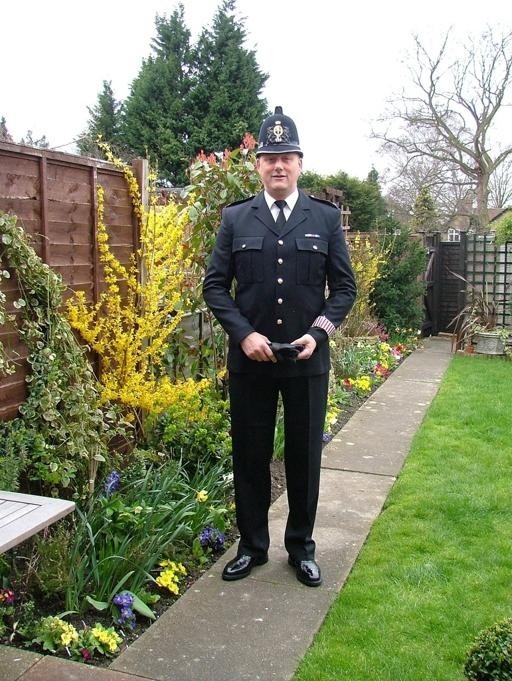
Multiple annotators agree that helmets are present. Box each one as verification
[256,106,303,158]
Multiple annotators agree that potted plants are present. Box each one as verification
[442,262,512,356]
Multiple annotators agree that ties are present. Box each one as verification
[275,200,287,232]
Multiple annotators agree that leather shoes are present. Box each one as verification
[288,554,322,586]
[222,553,267,581]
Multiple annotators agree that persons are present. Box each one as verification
[203,104,358,587]
